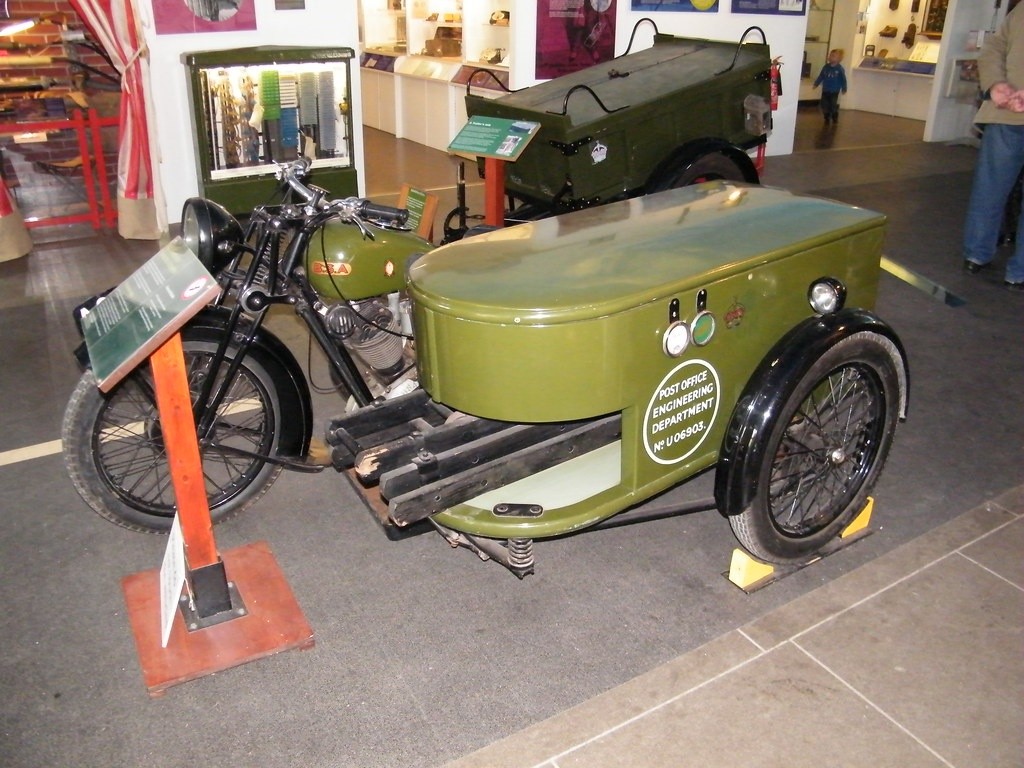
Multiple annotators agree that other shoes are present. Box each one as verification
[965,258,992,273]
[832,114,838,124]
[1004,277,1024,292]
[824,116,830,124]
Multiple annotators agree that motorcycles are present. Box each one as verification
[61,18,912,566]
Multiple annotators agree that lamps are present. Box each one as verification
[0,16,120,81]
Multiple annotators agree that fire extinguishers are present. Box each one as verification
[770,55,784,111]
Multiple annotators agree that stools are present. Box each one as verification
[36,153,106,215]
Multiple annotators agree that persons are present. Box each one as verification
[961,0,1024,289]
[812,49,847,128]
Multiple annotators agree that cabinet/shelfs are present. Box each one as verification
[357,0,521,162]
[799,0,836,82]
